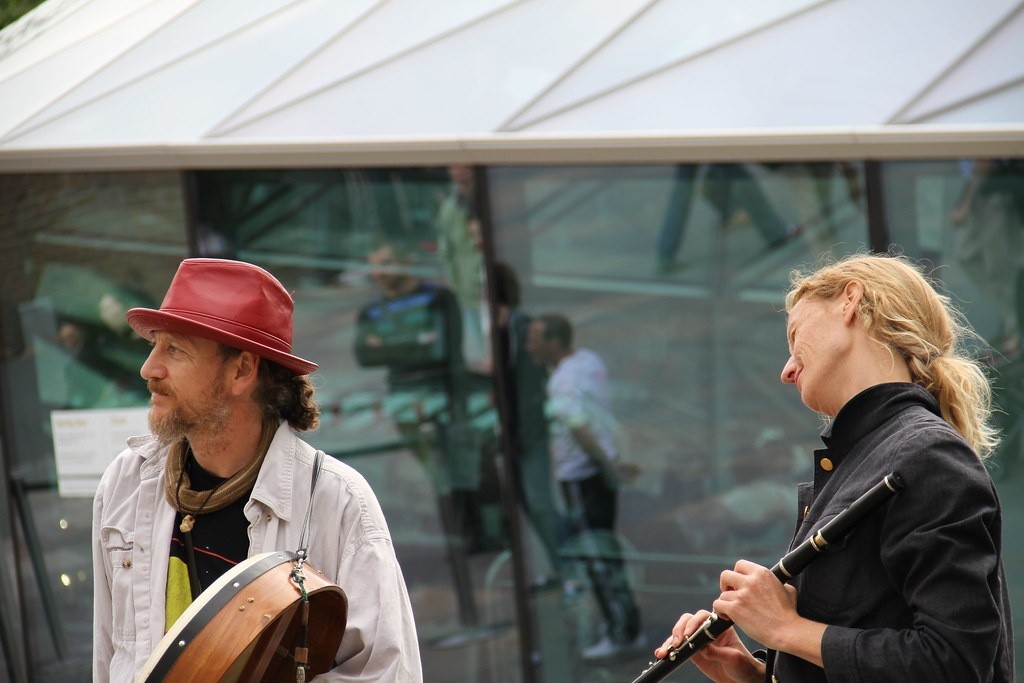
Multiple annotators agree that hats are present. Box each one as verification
[126,258,319,375]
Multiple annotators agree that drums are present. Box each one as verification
[131,549,349,683]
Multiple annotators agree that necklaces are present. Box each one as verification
[172,443,240,533]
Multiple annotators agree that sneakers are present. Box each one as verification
[579,634,651,665]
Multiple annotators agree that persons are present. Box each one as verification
[83,258,427,682]
[652,161,997,273]
[354,167,645,664]
[652,252,1016,682]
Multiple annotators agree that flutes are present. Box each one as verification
[630,470,907,683]
